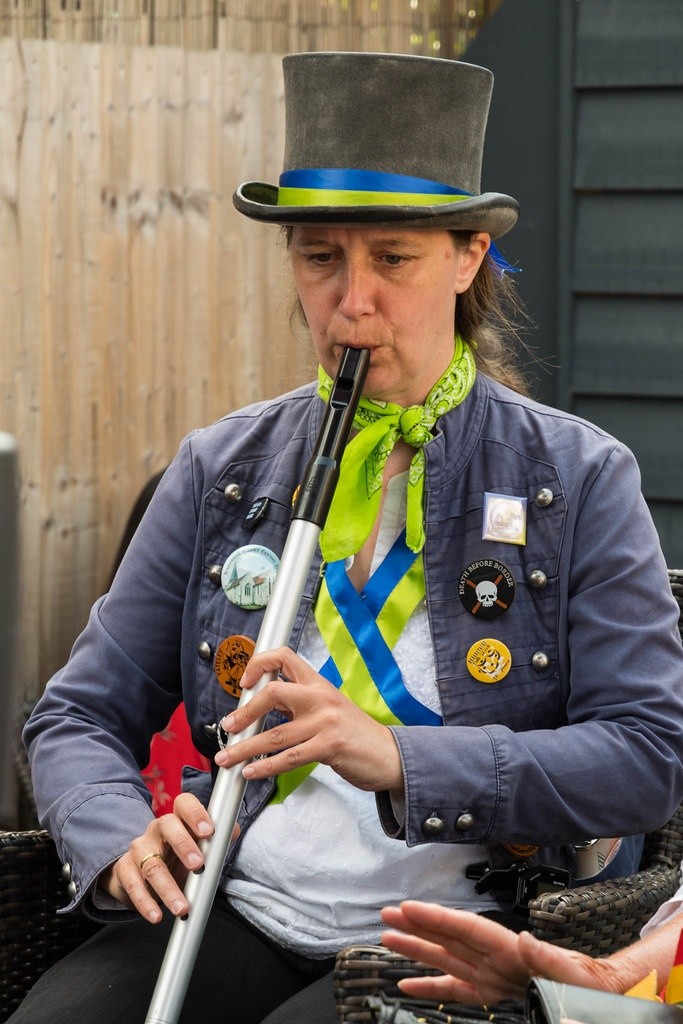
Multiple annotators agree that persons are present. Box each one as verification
[1,51,683,1022]
[380,885,683,1012]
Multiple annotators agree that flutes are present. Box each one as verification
[141,346,374,1023]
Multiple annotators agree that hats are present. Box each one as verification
[230,51,522,242]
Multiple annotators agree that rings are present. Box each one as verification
[139,852,163,866]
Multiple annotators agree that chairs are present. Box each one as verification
[0,567,681,1023]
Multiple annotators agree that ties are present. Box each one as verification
[316,332,479,562]
